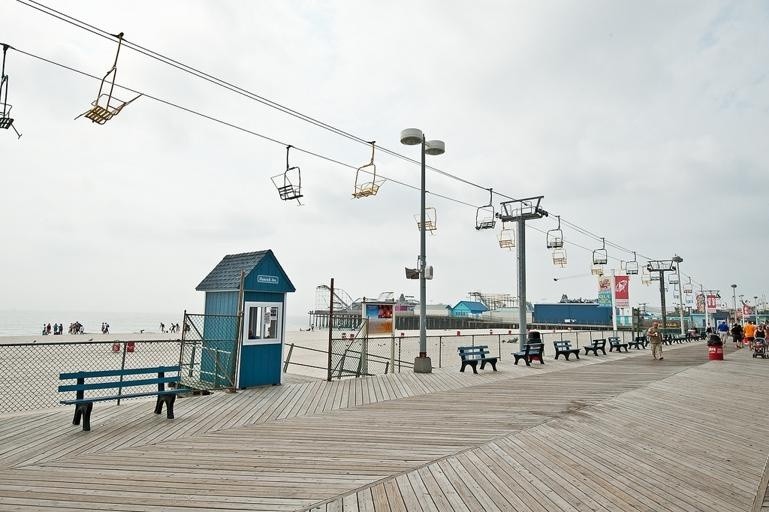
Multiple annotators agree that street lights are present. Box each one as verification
[674,254,686,337]
[729,280,759,323]
[400,128,446,374]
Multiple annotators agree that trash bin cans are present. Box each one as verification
[701,332,706,340]
[708,335,723,361]
[527,332,542,360]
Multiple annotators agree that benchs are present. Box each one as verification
[458,332,704,375]
[55,364,190,432]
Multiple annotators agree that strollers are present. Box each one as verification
[752,337,768,360]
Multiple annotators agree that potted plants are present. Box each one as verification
[707,332,724,361]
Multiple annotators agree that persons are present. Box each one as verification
[176,323,180,333]
[41,320,110,335]
[706,320,769,358]
[170,323,175,333]
[352,322,354,330]
[312,324,314,331]
[647,321,664,360]
[182,320,187,335]
[160,322,165,333]
[300,327,311,331]
